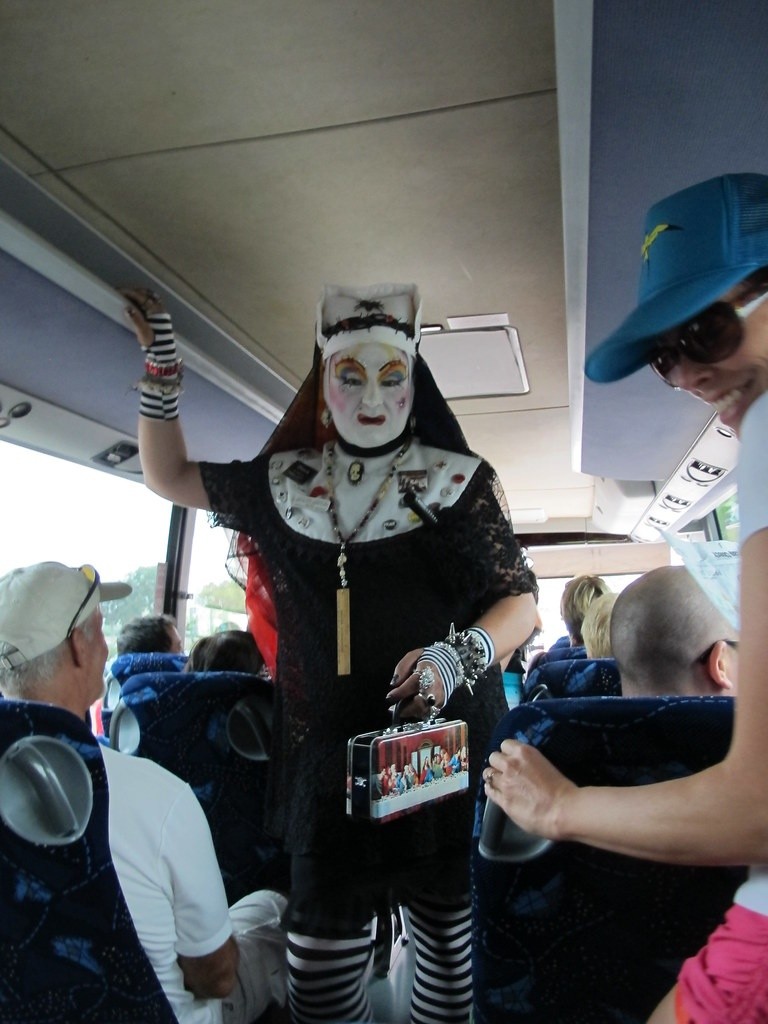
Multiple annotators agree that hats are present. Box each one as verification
[313,283,425,364]
[584,172,768,384]
[0,561,132,670]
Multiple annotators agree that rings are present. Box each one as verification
[487,772,498,788]
[416,667,435,689]
[430,706,440,717]
[152,292,161,300]
[419,693,436,707]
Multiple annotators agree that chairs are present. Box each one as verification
[521,659,623,703]
[119,672,293,909]
[0,697,180,1024]
[111,653,190,701]
[550,637,570,650]
[470,697,752,1024]
[545,647,588,664]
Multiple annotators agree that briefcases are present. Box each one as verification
[346,696,469,825]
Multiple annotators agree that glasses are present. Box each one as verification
[649,289,768,388]
[64,564,100,641]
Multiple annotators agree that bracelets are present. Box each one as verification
[140,361,184,393]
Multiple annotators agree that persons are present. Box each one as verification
[559,565,738,698]
[117,613,182,654]
[482,171,768,1024]
[0,563,291,1024]
[185,630,264,674]
[118,279,536,1024]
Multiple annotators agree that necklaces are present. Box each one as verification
[324,438,403,575]
